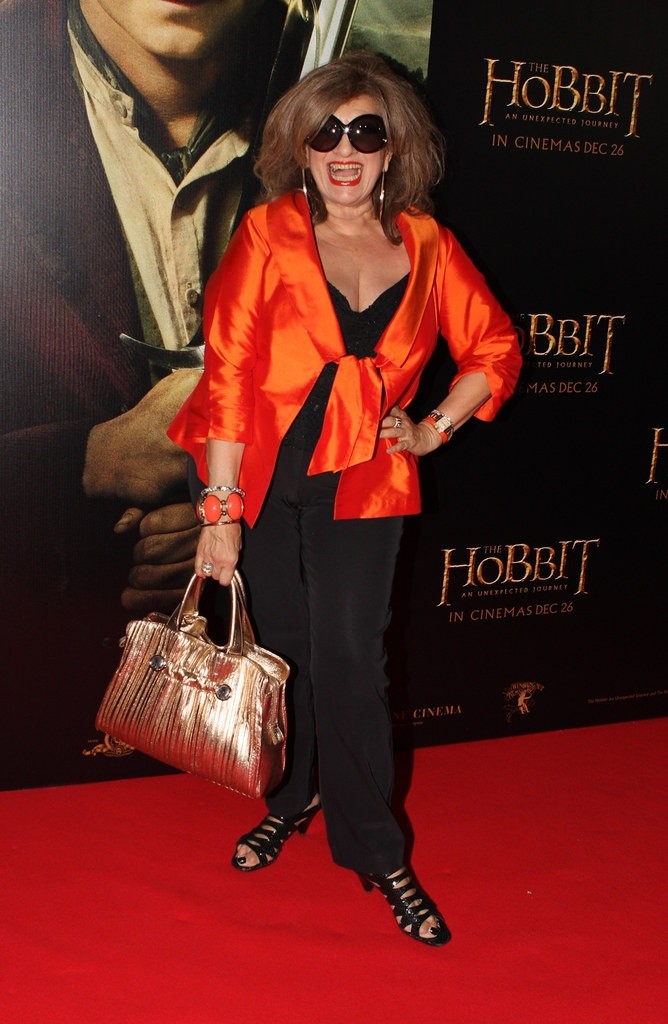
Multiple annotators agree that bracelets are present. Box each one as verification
[422,410,454,445]
[195,486,246,527]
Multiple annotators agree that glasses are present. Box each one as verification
[304,113,388,153]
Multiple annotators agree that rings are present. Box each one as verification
[201,561,213,573]
[393,418,403,428]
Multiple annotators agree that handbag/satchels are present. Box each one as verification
[96,569,289,798]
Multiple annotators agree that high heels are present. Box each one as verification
[361,864,451,948]
[231,805,321,870]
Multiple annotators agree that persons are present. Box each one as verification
[2,0,276,792]
[166,48,522,949]
